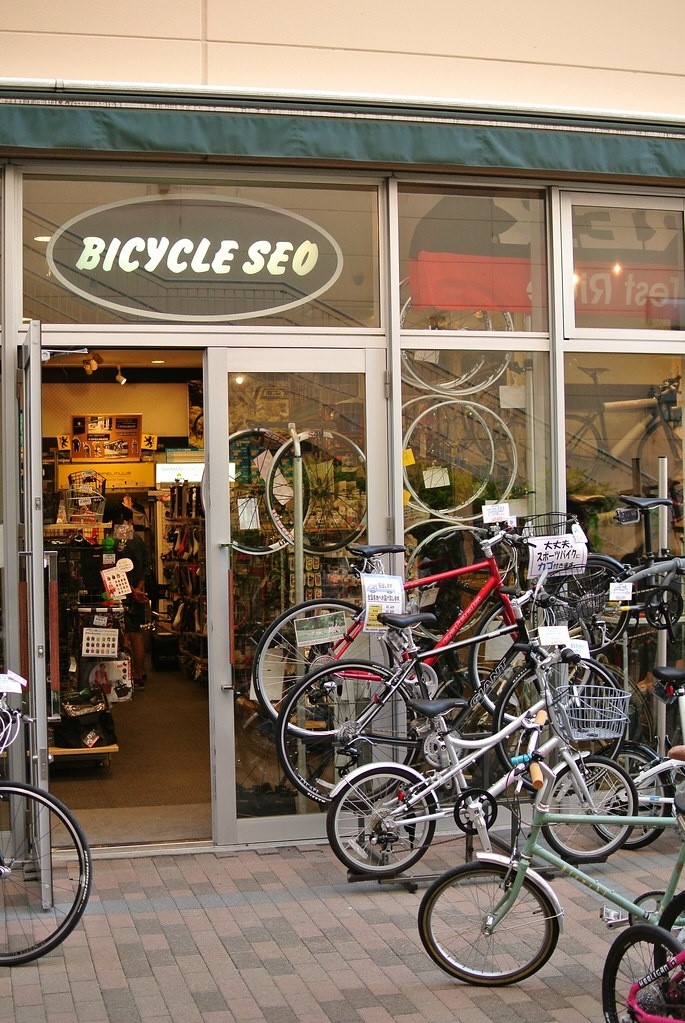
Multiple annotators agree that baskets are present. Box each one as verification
[532,565,607,622]
[555,686,631,739]
[515,511,576,561]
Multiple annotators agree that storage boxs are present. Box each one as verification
[78,654,132,703]
[69,412,144,463]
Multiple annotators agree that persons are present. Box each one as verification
[110,508,150,691]
[417,480,467,699]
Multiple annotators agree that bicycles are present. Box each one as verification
[0,708,93,967]
[418,709,685,1023]
[326,639,639,878]
[252,495,685,851]
[602,890,685,1023]
[564,367,683,495]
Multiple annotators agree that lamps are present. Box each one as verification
[84,349,104,375]
[115,364,127,386]
